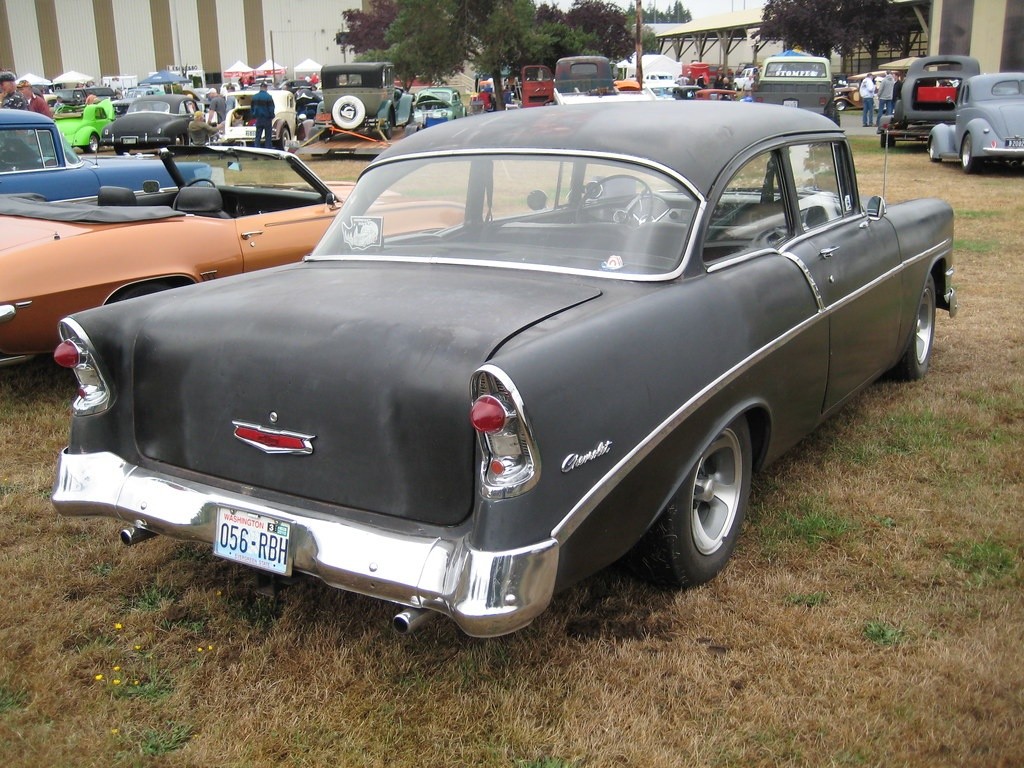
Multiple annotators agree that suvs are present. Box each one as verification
[750,57,840,127]
[833,70,888,111]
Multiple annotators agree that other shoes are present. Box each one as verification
[863,124,878,127]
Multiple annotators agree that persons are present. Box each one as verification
[298,73,320,84]
[85,94,98,104]
[673,71,709,88]
[714,67,733,101]
[206,88,227,124]
[54,95,66,112]
[250,83,275,160]
[76,81,94,88]
[115,90,126,113]
[742,74,758,96]
[189,111,224,144]
[226,82,235,92]
[16,80,54,157]
[0,71,30,144]
[859,70,903,127]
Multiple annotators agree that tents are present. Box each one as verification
[256,59,288,80]
[138,69,192,94]
[770,48,813,56]
[16,73,51,87]
[878,56,949,69]
[53,71,94,84]
[630,55,683,76]
[293,58,323,80]
[223,61,256,84]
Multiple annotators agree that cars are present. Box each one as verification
[734,68,758,91]
[876,54,981,147]
[0,108,213,204]
[1,146,500,356]
[927,72,1024,174]
[52,100,957,639]
[42,56,639,161]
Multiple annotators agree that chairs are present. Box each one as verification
[177,187,232,220]
[97,185,137,208]
[186,127,217,158]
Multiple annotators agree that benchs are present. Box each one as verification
[507,215,703,259]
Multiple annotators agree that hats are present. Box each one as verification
[16,79,32,88]
[194,111,204,119]
[0,71,16,82]
[261,82,268,88]
[205,88,217,95]
